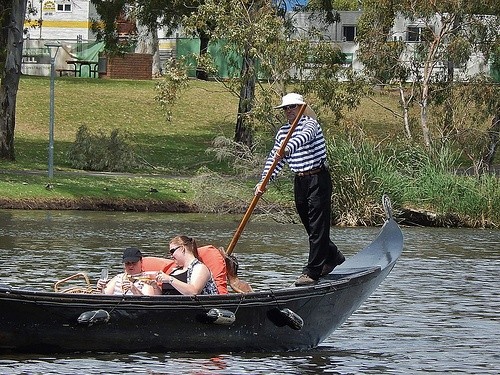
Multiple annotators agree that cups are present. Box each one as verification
[100,268,109,281]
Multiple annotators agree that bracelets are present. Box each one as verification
[169,276,175,285]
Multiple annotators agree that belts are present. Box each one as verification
[294,168,320,177]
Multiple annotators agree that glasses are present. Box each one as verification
[125,259,141,266]
[283,104,301,110]
[170,246,183,255]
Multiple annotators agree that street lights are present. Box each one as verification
[43,42,63,179]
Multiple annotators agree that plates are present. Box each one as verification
[132,276,157,282]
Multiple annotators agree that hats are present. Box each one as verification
[122,248,142,263]
[273,93,317,119]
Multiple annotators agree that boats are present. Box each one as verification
[1,195,408,360]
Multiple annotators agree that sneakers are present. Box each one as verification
[295,274,318,286]
[320,249,345,277]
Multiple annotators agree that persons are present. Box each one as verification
[156,235,220,295]
[256,93,345,286]
[103,248,157,296]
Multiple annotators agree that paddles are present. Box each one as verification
[225,103,307,256]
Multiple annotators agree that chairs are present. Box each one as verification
[143,244,229,295]
